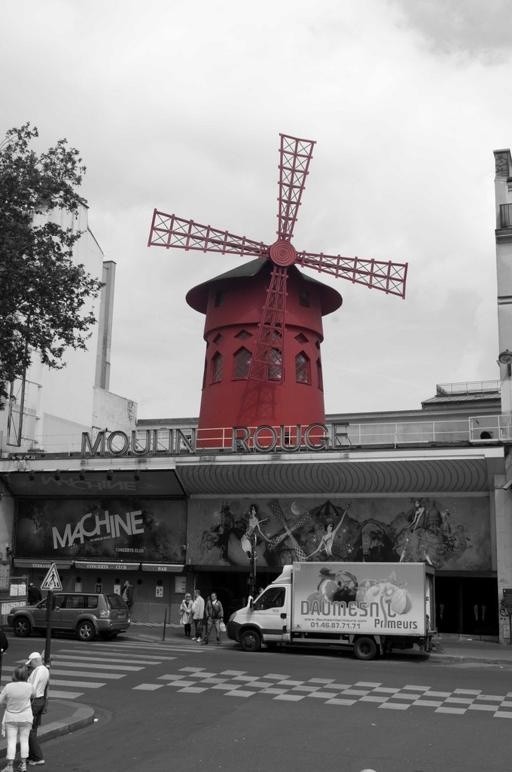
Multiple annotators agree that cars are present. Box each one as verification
[0,630,9,655]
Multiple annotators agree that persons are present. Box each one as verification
[0,665,35,772]
[240,504,275,561]
[120,580,133,625]
[0,627,9,653]
[302,503,350,561]
[179,589,224,646]
[25,651,50,766]
[399,500,434,566]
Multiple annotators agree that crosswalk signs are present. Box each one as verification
[40,562,65,592]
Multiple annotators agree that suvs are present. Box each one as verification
[6,593,131,640]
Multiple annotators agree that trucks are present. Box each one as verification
[225,561,437,661]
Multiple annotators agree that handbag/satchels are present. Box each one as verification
[177,609,184,617]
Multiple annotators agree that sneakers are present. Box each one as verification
[191,636,201,642]
[17,762,27,772]
[1,764,15,772]
[28,759,45,766]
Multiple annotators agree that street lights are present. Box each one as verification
[239,530,259,599]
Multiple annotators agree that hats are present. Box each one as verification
[25,652,41,665]
